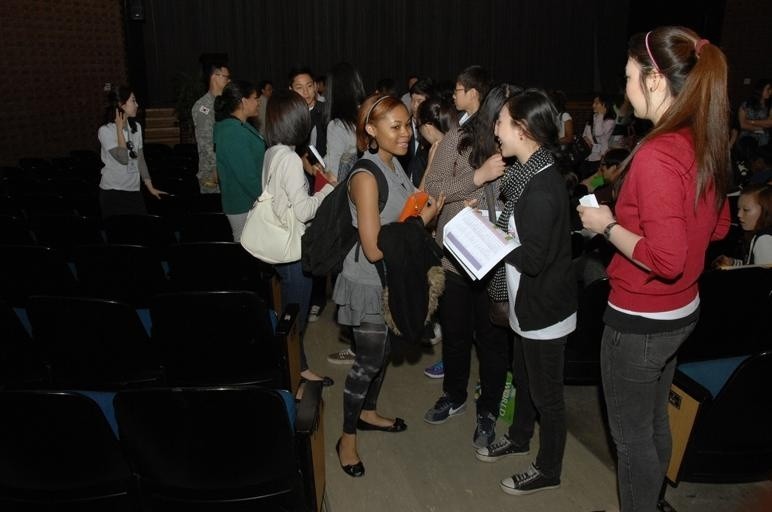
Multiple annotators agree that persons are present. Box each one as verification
[461,86,583,497]
[332,91,447,479]
[96,86,168,218]
[189,62,770,455]
[572,24,735,512]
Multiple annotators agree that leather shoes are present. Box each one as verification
[335,435,365,478]
[299,372,334,388]
[358,417,409,432]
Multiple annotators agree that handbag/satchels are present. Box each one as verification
[240,191,303,265]
[400,192,428,222]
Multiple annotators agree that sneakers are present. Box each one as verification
[424,393,467,426]
[499,461,562,496]
[424,358,444,379]
[326,350,355,365]
[470,408,499,449]
[475,432,531,464]
[308,300,327,323]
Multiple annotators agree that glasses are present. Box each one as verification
[453,87,472,95]
[214,73,230,80]
[601,158,607,166]
[414,120,431,131]
[125,139,137,159]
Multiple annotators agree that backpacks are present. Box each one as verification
[301,158,389,279]
